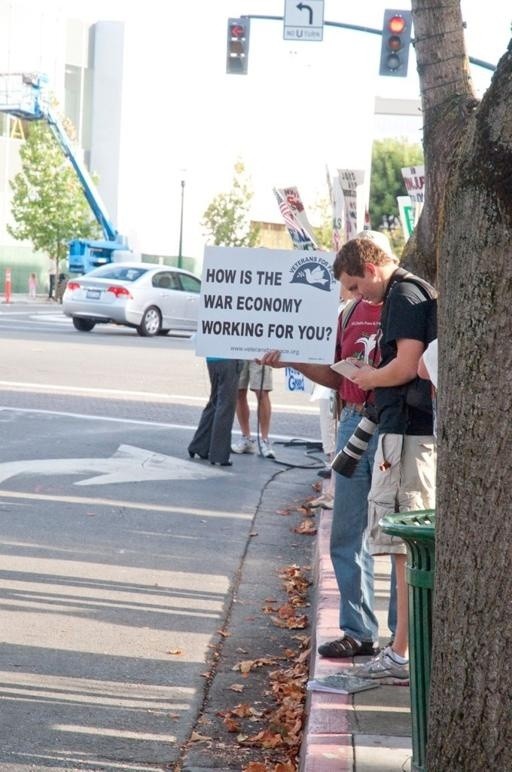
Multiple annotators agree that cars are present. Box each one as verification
[57,261,201,337]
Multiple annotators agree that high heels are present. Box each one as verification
[188,449,233,467]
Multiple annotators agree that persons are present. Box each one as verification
[329,236,438,679]
[235,360,278,460]
[187,357,240,467]
[415,336,439,402]
[29,272,38,299]
[254,230,399,657]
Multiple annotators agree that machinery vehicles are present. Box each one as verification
[0,69,140,276]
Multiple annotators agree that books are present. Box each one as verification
[329,357,378,384]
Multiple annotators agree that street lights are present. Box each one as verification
[177,178,186,268]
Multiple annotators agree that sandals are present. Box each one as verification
[317,636,394,659]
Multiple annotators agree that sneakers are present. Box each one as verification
[231,435,276,459]
[352,649,410,688]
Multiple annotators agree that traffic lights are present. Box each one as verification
[225,15,251,76]
[377,7,413,77]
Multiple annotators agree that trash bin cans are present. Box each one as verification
[378,508,436,772]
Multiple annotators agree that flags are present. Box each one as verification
[272,187,305,238]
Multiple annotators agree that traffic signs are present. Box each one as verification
[281,0,325,41]
